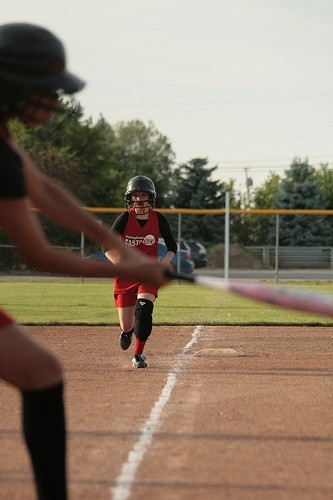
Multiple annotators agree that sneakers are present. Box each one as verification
[131,354,147,368]
[119,328,134,350]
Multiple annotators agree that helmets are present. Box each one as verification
[0,23,86,94]
[124,175,156,202]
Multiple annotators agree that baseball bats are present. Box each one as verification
[161,267,333,318]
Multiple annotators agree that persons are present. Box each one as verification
[0,22,176,500]
[103,174,177,368]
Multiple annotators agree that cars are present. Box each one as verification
[84,239,208,273]
